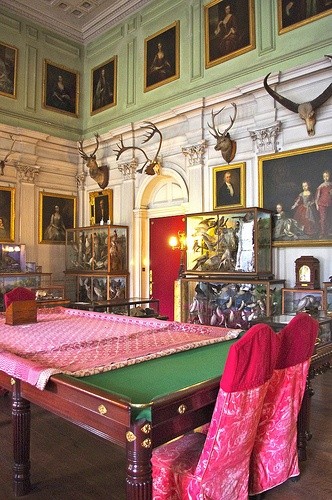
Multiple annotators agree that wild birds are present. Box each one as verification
[70,229,124,271]
[295,295,317,312]
[83,278,125,301]
[188,216,279,329]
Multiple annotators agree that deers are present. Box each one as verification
[207,103,237,163]
[113,120,164,176]
[78,133,108,190]
[0,135,16,176]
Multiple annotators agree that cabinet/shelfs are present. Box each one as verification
[0,244,71,312]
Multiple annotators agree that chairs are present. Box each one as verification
[150,314,319,500]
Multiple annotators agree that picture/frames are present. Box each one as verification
[212,162,246,210]
[277,0,332,35]
[41,58,81,118]
[90,55,118,116]
[0,186,16,243]
[259,142,332,247]
[89,189,113,225]
[38,191,78,245]
[204,0,256,69]
[0,42,19,99]
[144,20,180,93]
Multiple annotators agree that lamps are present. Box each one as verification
[168,231,187,278]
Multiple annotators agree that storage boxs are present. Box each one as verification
[185,207,274,279]
[181,279,286,330]
[64,226,130,315]
[5,300,36,325]
[281,282,332,317]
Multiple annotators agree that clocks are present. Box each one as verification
[294,256,321,290]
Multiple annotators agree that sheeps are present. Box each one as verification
[264,55,332,136]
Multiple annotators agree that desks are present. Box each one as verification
[0,305,309,500]
